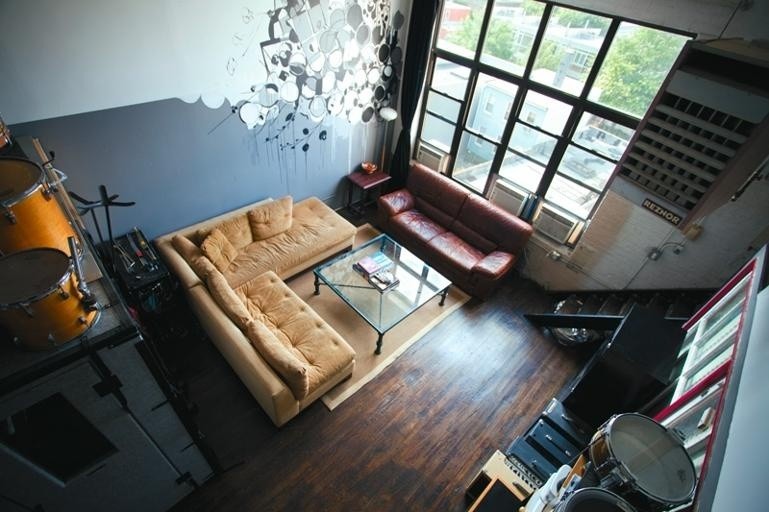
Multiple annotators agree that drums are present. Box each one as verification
[2,157,84,262]
[1,247,101,349]
[553,488,638,512]
[590,413,697,512]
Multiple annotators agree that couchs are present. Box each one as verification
[151,196,356,428]
[378,164,533,301]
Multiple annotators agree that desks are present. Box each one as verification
[346,170,392,214]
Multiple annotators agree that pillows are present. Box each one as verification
[199,229,238,272]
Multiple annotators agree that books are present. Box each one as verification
[351,251,400,295]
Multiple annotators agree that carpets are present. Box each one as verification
[284,224,471,411]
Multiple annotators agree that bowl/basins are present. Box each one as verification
[362,160,379,175]
